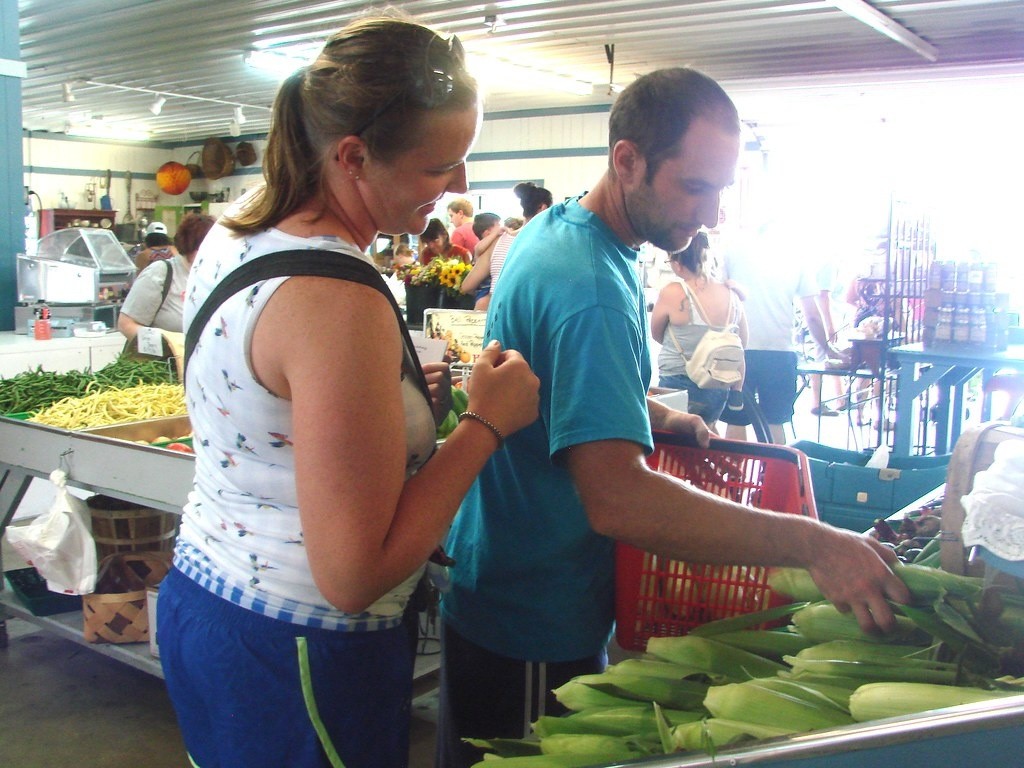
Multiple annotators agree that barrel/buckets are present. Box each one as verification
[85,493,176,562]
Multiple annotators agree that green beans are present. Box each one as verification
[0,351,188,432]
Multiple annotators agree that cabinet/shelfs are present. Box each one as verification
[0,328,132,378]
[36,208,117,238]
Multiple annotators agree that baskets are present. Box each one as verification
[82,552,169,644]
[231,142,257,166]
[618,429,819,655]
[203,139,236,180]
[185,151,202,176]
[939,420,1023,587]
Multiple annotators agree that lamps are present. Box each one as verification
[148,91,167,116]
[232,104,246,123]
[61,80,81,103]
[229,119,241,138]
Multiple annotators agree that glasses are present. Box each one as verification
[333,33,465,164]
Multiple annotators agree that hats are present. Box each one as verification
[147,222,168,235]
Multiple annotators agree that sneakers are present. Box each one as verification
[728,390,744,411]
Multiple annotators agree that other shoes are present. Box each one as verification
[811,405,838,417]
[857,417,871,426]
[836,400,858,412]
[873,419,895,431]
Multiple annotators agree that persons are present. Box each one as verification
[651,208,905,502]
[156,17,543,768]
[375,182,553,309]
[442,67,910,768]
[117,215,215,341]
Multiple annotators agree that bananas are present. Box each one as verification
[437,385,469,436]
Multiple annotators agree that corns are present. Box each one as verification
[470,561,1024,768]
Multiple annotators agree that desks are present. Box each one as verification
[887,337,1024,467]
[0,460,441,729]
[796,361,931,457]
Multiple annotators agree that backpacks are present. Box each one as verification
[666,278,746,391]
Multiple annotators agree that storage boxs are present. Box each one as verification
[3,567,85,617]
[149,584,164,656]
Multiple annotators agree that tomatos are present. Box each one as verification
[166,442,193,452]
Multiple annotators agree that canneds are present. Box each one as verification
[935,289,987,343]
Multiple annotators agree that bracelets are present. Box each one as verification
[458,412,504,441]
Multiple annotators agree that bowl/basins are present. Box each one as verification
[73,320,107,338]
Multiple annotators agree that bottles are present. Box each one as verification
[33,300,51,340]
